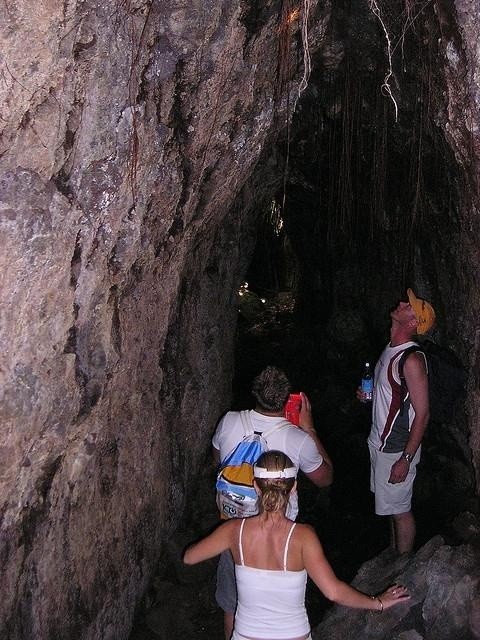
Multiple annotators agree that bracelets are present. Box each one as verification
[369,597,386,617]
[401,452,414,463]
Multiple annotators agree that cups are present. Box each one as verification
[283,393,302,427]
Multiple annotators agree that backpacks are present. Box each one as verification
[216,410,291,518]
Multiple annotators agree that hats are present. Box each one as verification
[407,288,436,335]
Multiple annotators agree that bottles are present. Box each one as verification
[360,362,374,401]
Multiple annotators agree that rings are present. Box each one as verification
[392,590,396,593]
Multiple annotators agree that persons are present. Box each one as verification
[182,449,413,640]
[210,364,335,640]
[354,285,437,556]
